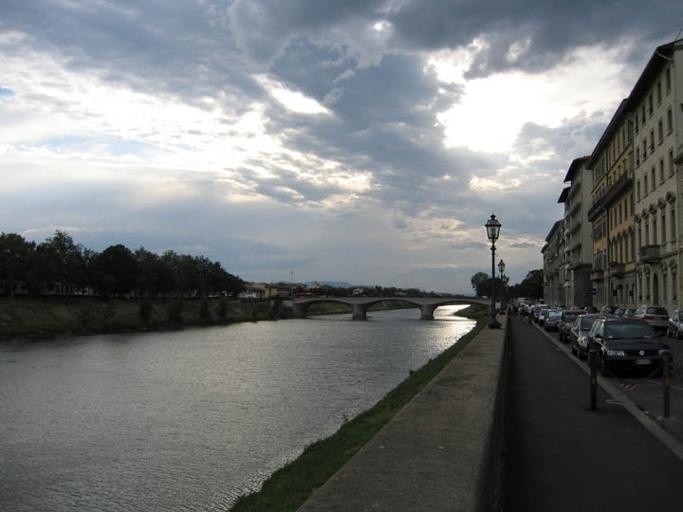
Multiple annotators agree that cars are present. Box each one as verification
[518,298,683,374]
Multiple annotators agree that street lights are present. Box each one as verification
[498,258,510,315]
[484,214,503,328]
